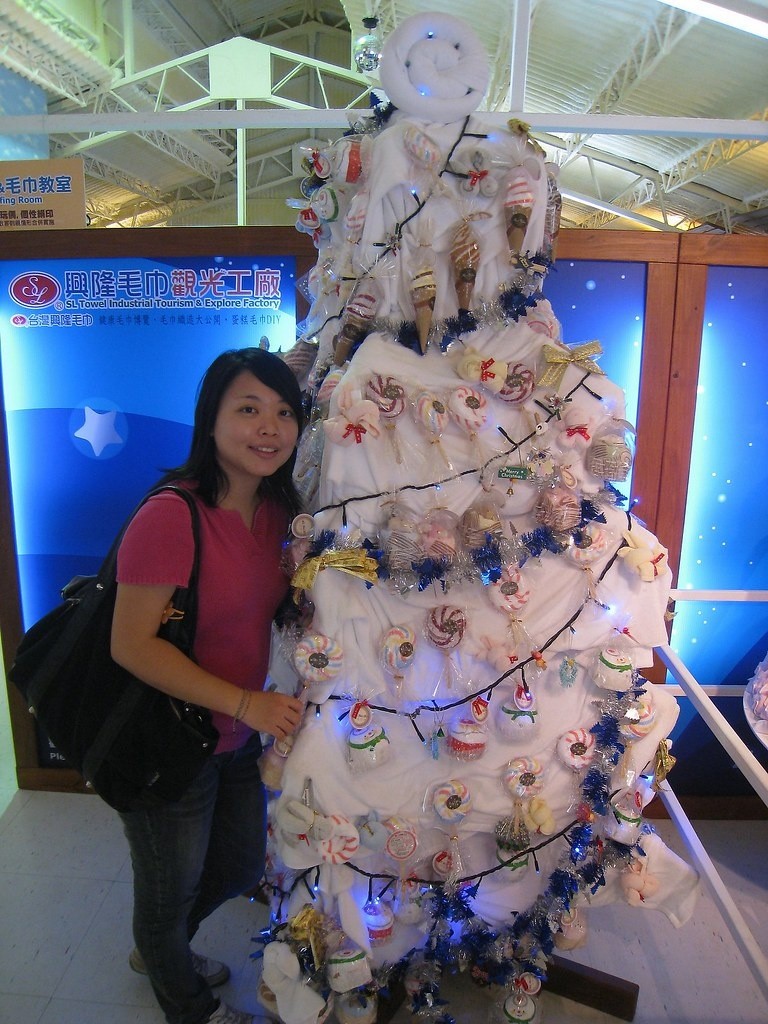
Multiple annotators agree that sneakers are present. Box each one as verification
[129,945,231,986]
[204,995,279,1024]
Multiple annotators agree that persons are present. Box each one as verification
[109,347,312,1024]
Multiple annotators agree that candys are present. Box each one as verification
[275,365,659,882]
[307,173,562,365]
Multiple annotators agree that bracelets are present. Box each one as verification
[232,687,252,733]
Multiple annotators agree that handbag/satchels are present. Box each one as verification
[12,484,226,821]
